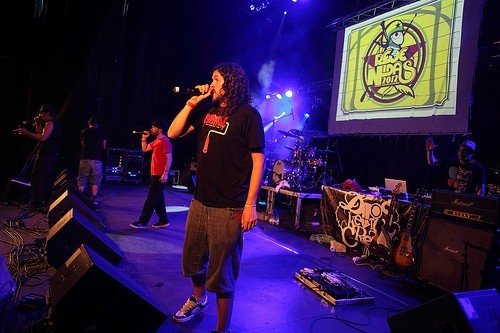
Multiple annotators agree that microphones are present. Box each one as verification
[133,131,147,135]
[173,87,208,96]
[292,109,294,120]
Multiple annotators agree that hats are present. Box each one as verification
[458,140,477,154]
[37,105,54,113]
[153,119,168,134]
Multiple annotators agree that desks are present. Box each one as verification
[321,185,432,267]
[261,185,322,232]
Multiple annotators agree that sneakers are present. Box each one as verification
[172,294,210,322]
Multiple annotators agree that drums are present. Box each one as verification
[306,159,321,170]
[292,149,307,160]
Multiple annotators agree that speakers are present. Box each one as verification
[415,215,500,293]
[44,167,168,333]
[387,288,500,333]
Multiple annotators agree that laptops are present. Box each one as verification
[384,178,407,193]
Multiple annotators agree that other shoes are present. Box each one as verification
[130,221,147,228]
[93,197,99,205]
[152,221,170,228]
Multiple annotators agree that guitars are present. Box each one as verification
[394,187,424,268]
[368,183,402,267]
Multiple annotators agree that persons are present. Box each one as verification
[168,62,265,333]
[130,122,173,229]
[16,104,61,208]
[425,136,487,197]
[76,117,105,204]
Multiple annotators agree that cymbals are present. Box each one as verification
[289,129,305,136]
[283,145,308,152]
[277,129,300,139]
[316,149,336,153]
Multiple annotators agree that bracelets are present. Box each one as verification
[165,170,169,172]
[142,140,146,142]
[427,149,434,152]
[245,204,256,207]
[185,100,197,109]
